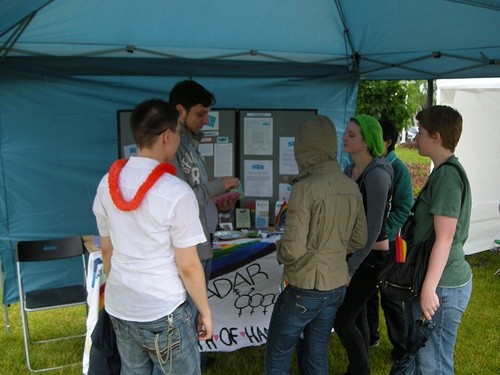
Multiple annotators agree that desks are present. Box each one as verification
[82,227,303,375]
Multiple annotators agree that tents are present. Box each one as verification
[429,77,500,258]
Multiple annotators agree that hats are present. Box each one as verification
[350,114,384,157]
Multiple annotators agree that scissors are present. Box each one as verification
[213,243,228,247]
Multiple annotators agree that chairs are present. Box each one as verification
[15,237,89,372]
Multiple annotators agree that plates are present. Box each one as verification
[214,231,243,240]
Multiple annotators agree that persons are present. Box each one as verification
[92,97,215,375]
[401,104,474,375]
[148,78,241,329]
[264,113,369,375]
[341,113,396,375]
[368,111,417,374]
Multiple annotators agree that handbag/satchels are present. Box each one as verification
[400,214,415,240]
[376,240,436,355]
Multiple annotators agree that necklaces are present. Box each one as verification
[107,158,177,211]
